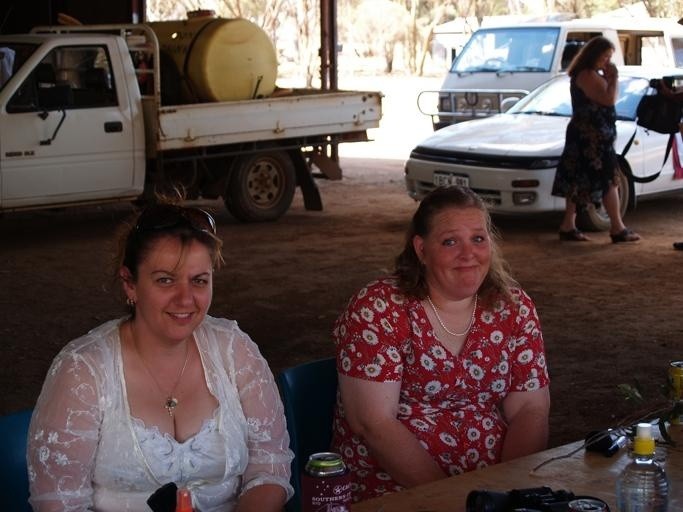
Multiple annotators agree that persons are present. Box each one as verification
[332,185,550,499]
[552,38,642,243]
[25,199,292,512]
[652,18,683,249]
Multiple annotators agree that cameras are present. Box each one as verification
[650,78,674,93]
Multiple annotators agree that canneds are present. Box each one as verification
[668,361,683,425]
[306,452,346,477]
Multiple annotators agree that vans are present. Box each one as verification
[413,14,683,137]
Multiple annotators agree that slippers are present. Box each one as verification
[610,227,640,243]
[560,229,590,242]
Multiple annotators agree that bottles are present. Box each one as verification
[174,490,194,511]
[615,422,672,512]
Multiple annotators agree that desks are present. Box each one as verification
[347,421,683,512]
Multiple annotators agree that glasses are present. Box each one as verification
[137,205,217,236]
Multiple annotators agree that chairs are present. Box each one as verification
[0,406,37,512]
[278,355,343,511]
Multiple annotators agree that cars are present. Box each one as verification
[392,62,683,233]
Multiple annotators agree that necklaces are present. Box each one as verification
[129,328,190,416]
[425,295,478,336]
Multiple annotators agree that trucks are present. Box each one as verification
[0,18,386,231]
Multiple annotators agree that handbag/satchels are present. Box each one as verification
[636,90,683,134]
